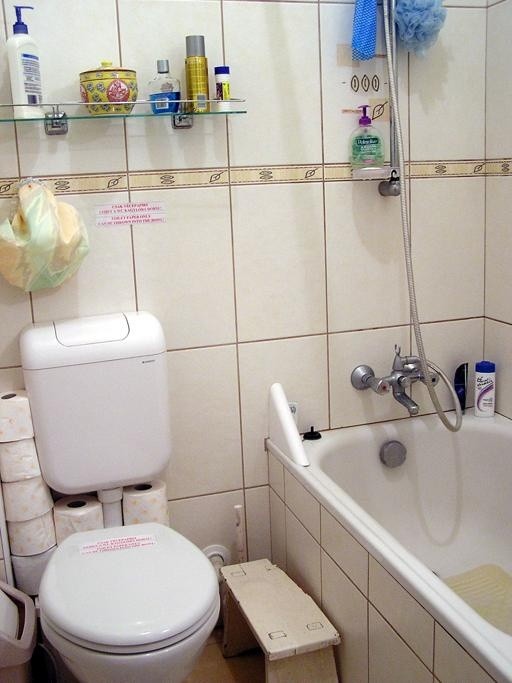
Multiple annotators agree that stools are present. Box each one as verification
[215,557,342,682]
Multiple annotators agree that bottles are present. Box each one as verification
[214,65,231,100]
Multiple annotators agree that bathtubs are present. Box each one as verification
[265,408,512,683]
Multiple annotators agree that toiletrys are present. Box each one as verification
[214,64,232,110]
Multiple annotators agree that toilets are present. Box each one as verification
[20,312,223,681]
